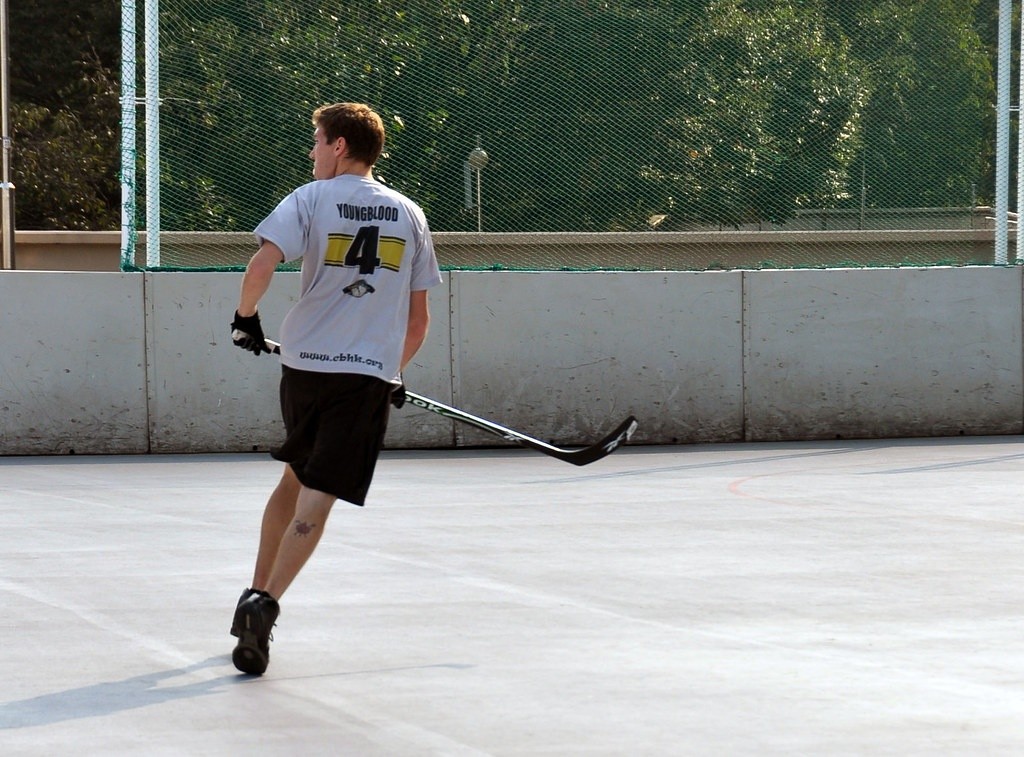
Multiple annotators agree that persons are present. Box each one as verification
[231,102,442,676]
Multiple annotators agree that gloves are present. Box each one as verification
[231,309,271,356]
[390,372,406,409]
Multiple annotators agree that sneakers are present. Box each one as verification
[230,587,280,676]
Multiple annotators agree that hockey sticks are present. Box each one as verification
[225,325,643,473]
